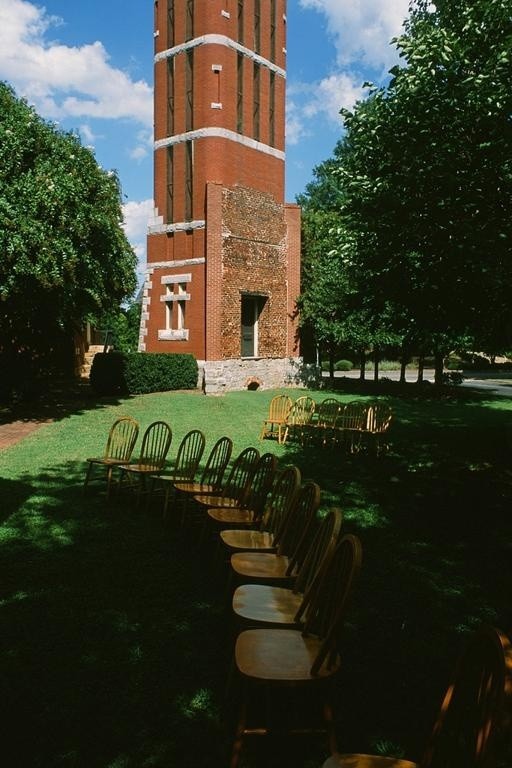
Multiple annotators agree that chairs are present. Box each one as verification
[86,418,172,510]
[224,510,342,691]
[230,482,321,612]
[220,534,362,768]
[323,624,510,768]
[259,394,393,458]
[142,429,280,567]
